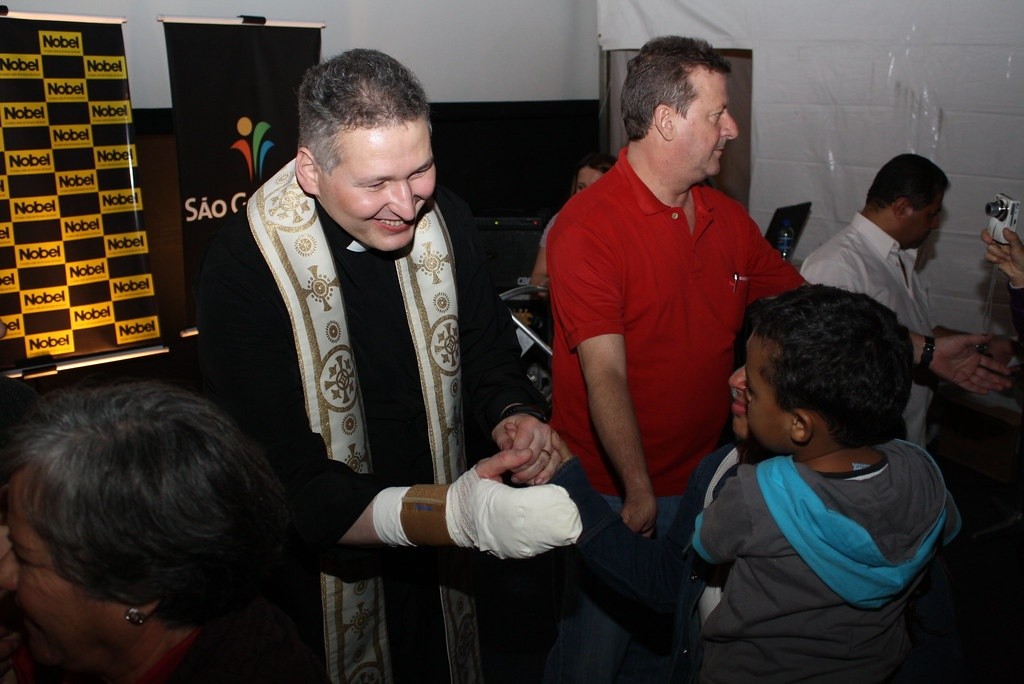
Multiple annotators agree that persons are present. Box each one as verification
[506,365,776,684]
[798,154,949,452]
[543,37,1012,684]
[199,51,586,684]
[0,319,332,684]
[690,284,964,684]
[529,154,616,371]
[981,227,1024,344]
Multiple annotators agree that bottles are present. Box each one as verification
[778,220,794,260]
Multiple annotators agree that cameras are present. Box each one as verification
[985,192,1020,244]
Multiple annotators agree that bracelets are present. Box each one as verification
[918,337,936,370]
[976,338,990,353]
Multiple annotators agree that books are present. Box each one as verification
[499,285,548,302]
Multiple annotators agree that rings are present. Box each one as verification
[542,449,551,458]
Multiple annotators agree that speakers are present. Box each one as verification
[472,214,546,287]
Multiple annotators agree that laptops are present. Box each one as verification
[764,201,812,261]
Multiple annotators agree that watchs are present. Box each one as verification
[502,406,549,423]
